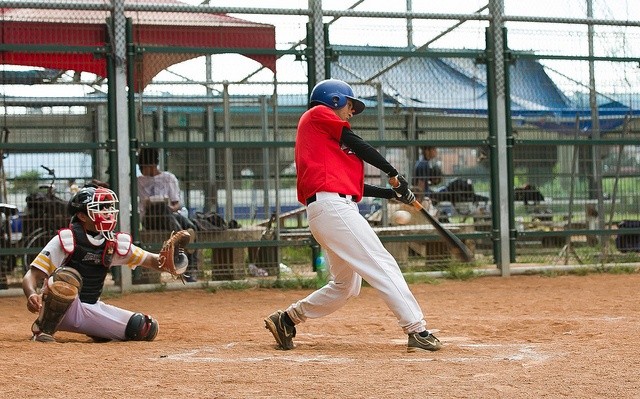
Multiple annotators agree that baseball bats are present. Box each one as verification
[388,177,474,262]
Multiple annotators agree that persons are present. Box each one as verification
[412,179,439,223]
[64,178,77,202]
[263,79,444,351]
[412,146,437,204]
[136,147,197,273]
[23,186,160,341]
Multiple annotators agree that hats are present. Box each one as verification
[138,147,159,165]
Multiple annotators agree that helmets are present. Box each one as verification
[310,79,365,115]
[67,184,120,242]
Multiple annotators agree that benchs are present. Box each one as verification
[132,230,262,285]
[373,224,475,268]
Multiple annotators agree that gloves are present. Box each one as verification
[396,189,415,204]
[392,175,408,195]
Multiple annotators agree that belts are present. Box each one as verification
[307,194,355,205]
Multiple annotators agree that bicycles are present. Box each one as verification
[0,165,59,284]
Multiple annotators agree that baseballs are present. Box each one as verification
[393,210,411,224]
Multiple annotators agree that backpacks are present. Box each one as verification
[190,212,226,231]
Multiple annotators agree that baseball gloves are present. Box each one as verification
[158,230,191,276]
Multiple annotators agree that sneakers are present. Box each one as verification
[264,310,296,350]
[183,272,196,282]
[87,334,112,343]
[31,322,54,340]
[407,329,444,353]
[184,228,196,254]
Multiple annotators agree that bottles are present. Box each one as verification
[316,250,328,285]
[201,249,214,281]
